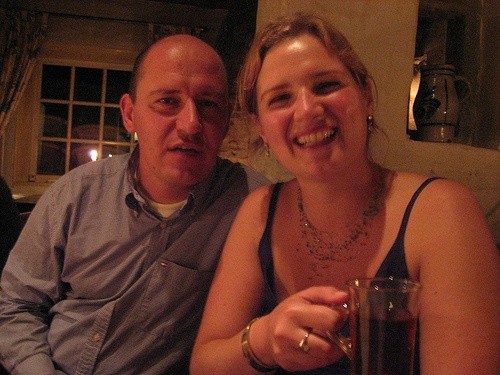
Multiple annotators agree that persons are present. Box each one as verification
[0,34,275,375]
[189,11,500,374]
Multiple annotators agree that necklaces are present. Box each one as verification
[297,160,387,282]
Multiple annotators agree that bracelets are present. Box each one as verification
[241,316,281,373]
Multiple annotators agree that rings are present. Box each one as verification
[297,330,311,352]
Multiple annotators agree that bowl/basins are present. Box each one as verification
[43,114,67,137]
[73,146,126,167]
[73,123,126,142]
[39,143,59,167]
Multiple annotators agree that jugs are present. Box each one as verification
[413,64,471,143]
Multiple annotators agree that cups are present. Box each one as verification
[325,278,418,375]
